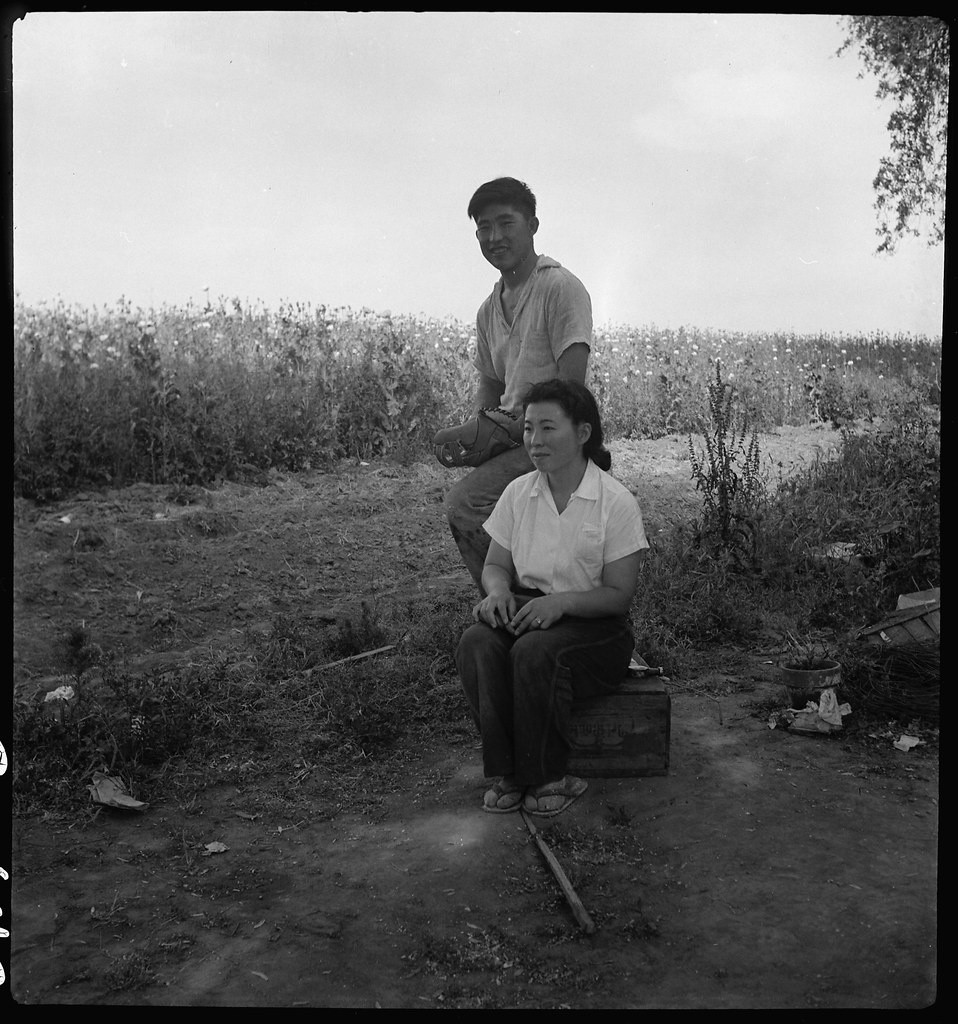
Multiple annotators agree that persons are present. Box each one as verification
[430,177,593,598]
[457,378,649,815]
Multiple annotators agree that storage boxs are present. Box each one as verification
[565,650,671,777]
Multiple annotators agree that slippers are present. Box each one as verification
[481,781,525,813]
[524,771,588,816]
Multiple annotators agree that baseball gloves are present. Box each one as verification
[431,408,522,469]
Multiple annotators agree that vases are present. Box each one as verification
[781,659,842,709]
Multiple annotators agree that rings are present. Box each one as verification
[535,617,541,624]
